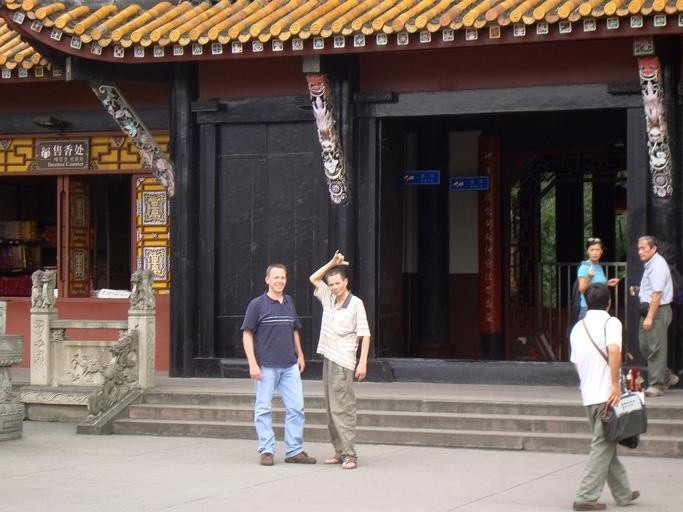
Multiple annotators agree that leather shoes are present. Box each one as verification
[572,500,606,512]
[616,489,640,506]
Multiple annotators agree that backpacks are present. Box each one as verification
[570,278,586,315]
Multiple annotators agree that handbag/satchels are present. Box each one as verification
[600,394,648,450]
[636,300,653,317]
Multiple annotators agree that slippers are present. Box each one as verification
[338,455,358,469]
[323,454,341,465]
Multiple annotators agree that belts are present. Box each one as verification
[658,302,670,307]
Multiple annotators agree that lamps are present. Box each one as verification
[34,115,69,132]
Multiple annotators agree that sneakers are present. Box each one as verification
[644,383,664,397]
[285,450,317,466]
[259,452,275,466]
[663,373,679,394]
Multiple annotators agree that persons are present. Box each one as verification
[576,236,620,322]
[629,236,680,396]
[569,283,641,509]
[240,262,316,463]
[309,250,371,470]
